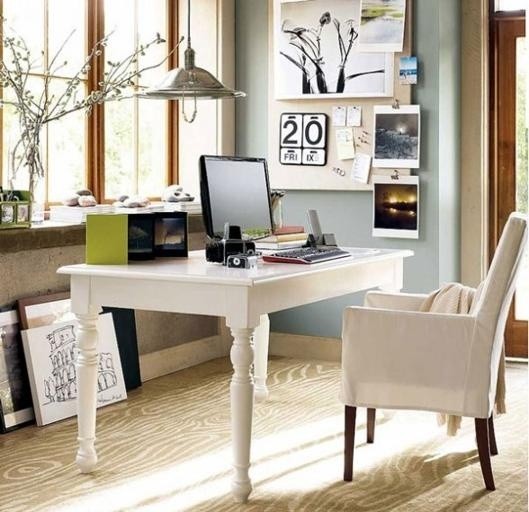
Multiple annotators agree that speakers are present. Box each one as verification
[244,242,256,256]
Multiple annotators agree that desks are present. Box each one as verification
[56,246,414,505]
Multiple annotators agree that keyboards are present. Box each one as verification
[262,245,350,264]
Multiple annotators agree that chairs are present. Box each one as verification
[341,211,529,491]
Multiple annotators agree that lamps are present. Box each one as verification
[135,0,246,123]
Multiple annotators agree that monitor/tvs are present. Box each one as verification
[200,155,275,252]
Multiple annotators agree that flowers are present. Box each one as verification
[0,15,184,200]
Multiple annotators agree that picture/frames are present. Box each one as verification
[17,291,71,329]
[0,304,36,434]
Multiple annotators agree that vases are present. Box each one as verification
[0,201,32,230]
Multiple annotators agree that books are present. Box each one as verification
[272,225,304,235]
[253,241,310,250]
[257,234,309,243]
